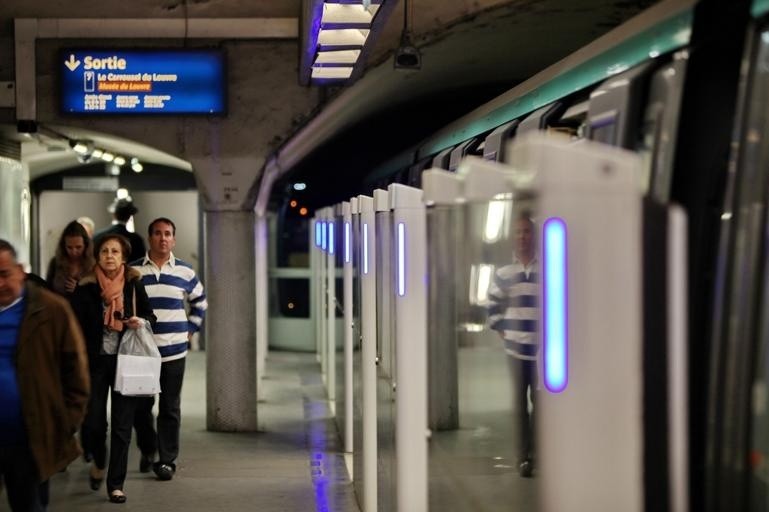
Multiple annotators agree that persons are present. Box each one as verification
[487,214,545,479]
[70,232,157,505]
[43,220,98,473]
[0,237,91,511]
[92,197,145,263]
[128,216,208,483]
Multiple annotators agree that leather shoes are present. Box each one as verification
[140,451,174,479]
[110,489,126,503]
[89,464,103,491]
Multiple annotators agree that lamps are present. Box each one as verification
[293,1,392,93]
